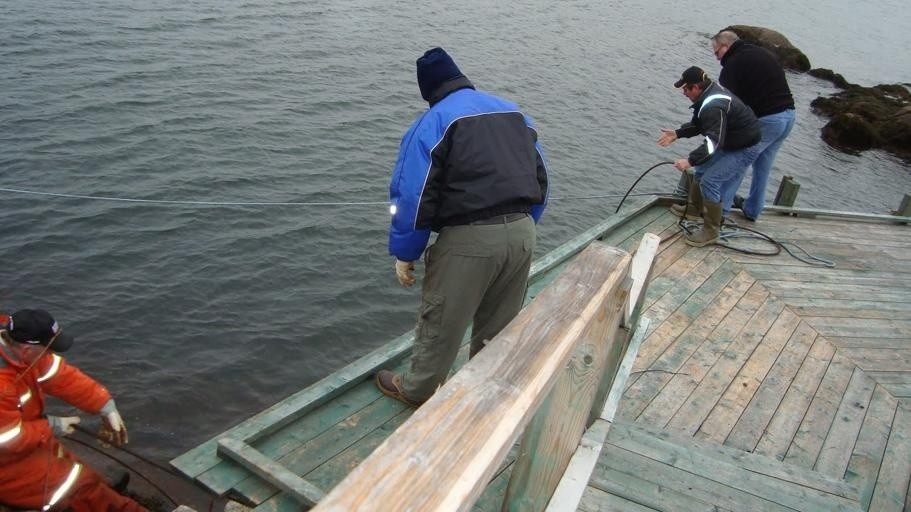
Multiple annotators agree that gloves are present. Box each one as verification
[95,408,129,451]
[40,414,81,440]
[391,255,419,288]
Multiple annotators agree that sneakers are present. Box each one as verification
[732,192,757,223]
[375,368,430,411]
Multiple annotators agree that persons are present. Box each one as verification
[372,47,552,412]
[1,308,150,511]
[657,65,761,248]
[710,30,796,229]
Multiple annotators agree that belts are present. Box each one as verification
[468,210,529,226]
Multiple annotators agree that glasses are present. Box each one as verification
[711,44,727,56]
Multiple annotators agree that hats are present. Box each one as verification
[670,65,710,90]
[412,44,476,110]
[1,309,75,354]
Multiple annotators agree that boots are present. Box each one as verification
[682,199,724,248]
[668,177,702,222]
